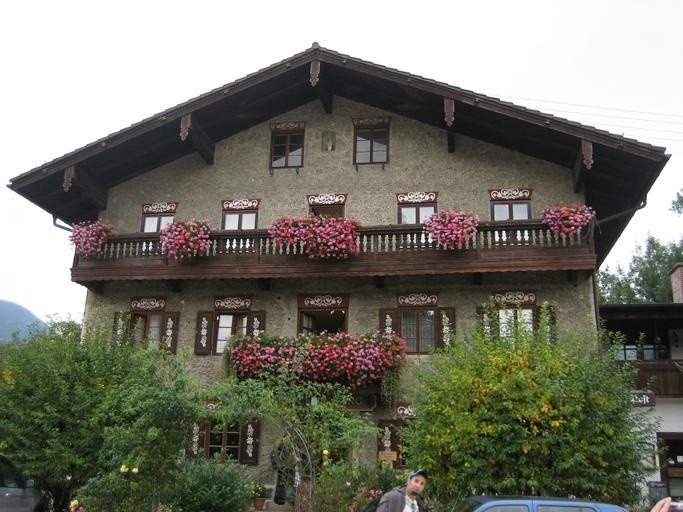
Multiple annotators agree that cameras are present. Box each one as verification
[668,502,683,512]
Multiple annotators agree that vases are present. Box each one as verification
[254,498,265,510]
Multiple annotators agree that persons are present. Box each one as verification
[375,468,429,512]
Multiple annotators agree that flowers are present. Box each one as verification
[266,213,359,260]
[540,204,597,238]
[70,217,114,258]
[225,329,410,413]
[425,208,481,250]
[156,216,213,264]
[252,481,266,497]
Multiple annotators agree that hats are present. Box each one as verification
[409,469,427,480]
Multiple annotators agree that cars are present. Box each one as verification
[0,454,51,512]
[453,495,630,512]
